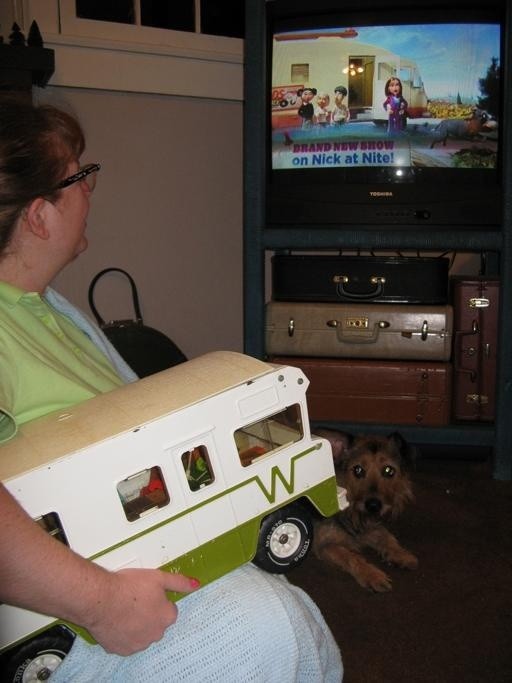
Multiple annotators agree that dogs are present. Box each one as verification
[309,425,419,596]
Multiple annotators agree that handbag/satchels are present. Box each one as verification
[88,267,188,378]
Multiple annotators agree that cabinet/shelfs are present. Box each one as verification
[243,0,512,481]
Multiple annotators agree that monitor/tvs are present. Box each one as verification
[242,0,512,249]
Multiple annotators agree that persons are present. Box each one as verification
[413,118,482,139]
[296,86,316,131]
[1,104,348,681]
[381,76,409,132]
[330,84,351,123]
[314,92,333,125]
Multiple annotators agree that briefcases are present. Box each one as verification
[268,356,454,428]
[271,255,451,304]
[455,278,500,423]
[265,304,453,362]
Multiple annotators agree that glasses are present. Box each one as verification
[52,163,101,191]
